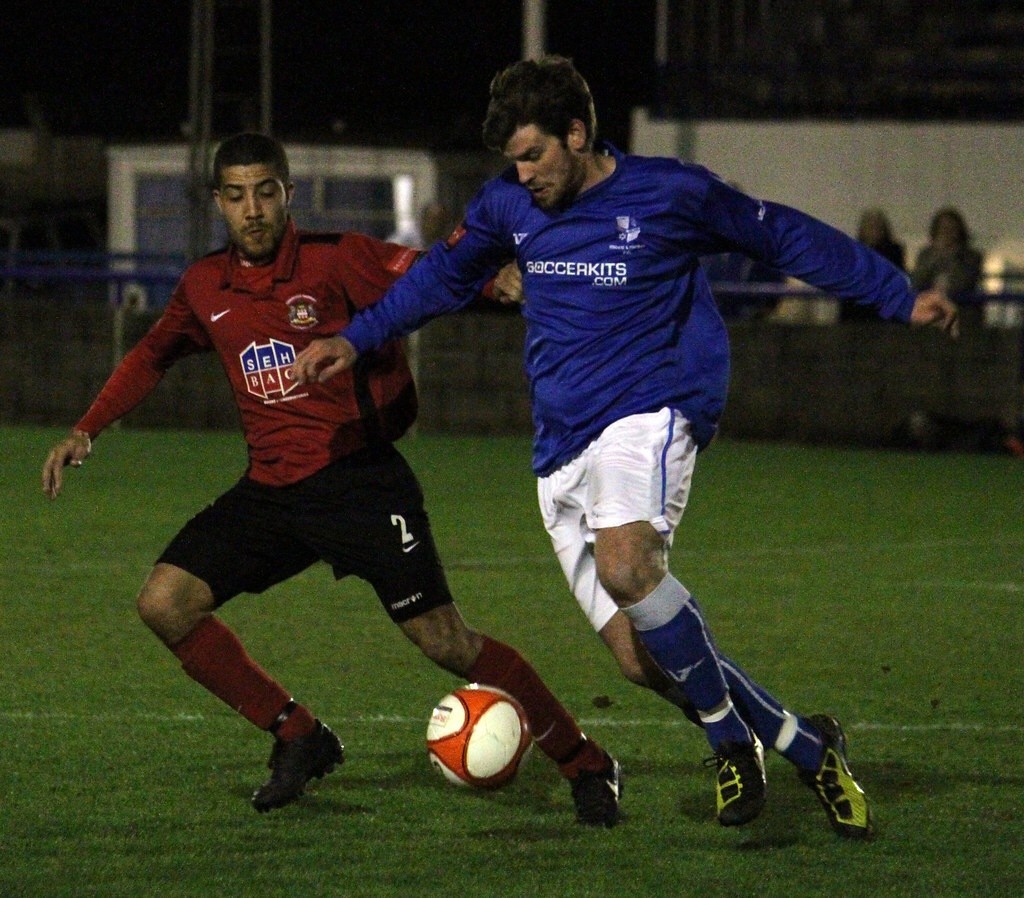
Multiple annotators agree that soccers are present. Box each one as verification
[426,682,535,792]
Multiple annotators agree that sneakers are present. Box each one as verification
[567,747,624,832]
[791,714,875,839]
[249,721,344,812]
[712,729,769,830]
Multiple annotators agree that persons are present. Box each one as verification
[293,58,959,838]
[913,210,984,326]
[837,208,906,318]
[40,134,622,830]
